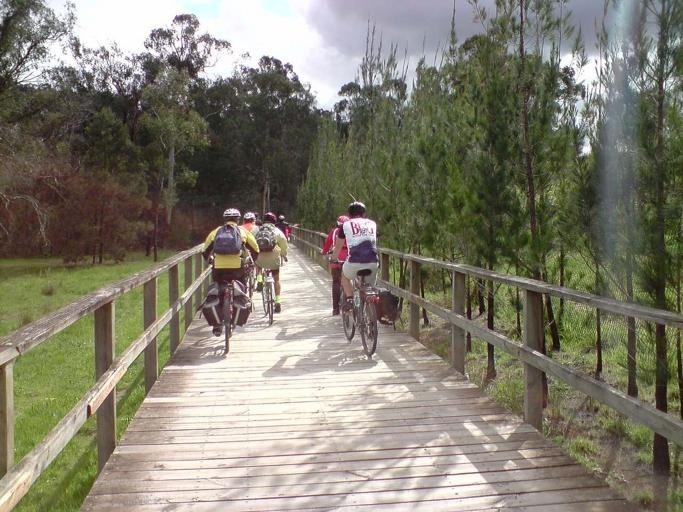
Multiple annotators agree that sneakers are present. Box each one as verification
[257,282,263,291]
[274,302,282,313]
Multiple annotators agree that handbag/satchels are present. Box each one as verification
[201,282,223,326]
[232,279,253,326]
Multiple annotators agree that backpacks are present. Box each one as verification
[214,223,241,255]
[255,223,277,250]
[377,290,399,331]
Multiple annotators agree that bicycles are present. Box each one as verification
[319,247,348,315]
[204,258,254,353]
[245,253,263,297]
[330,259,377,356]
[250,250,289,325]
[279,235,290,267]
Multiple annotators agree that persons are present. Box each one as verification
[254,211,288,313]
[254,212,261,226]
[274,214,292,262]
[201,208,260,337]
[239,212,260,281]
[330,200,379,338]
[318,215,348,315]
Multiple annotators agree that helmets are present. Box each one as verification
[223,208,241,217]
[348,201,366,211]
[264,212,277,222]
[279,215,285,220]
[244,211,256,220]
[337,215,349,225]
